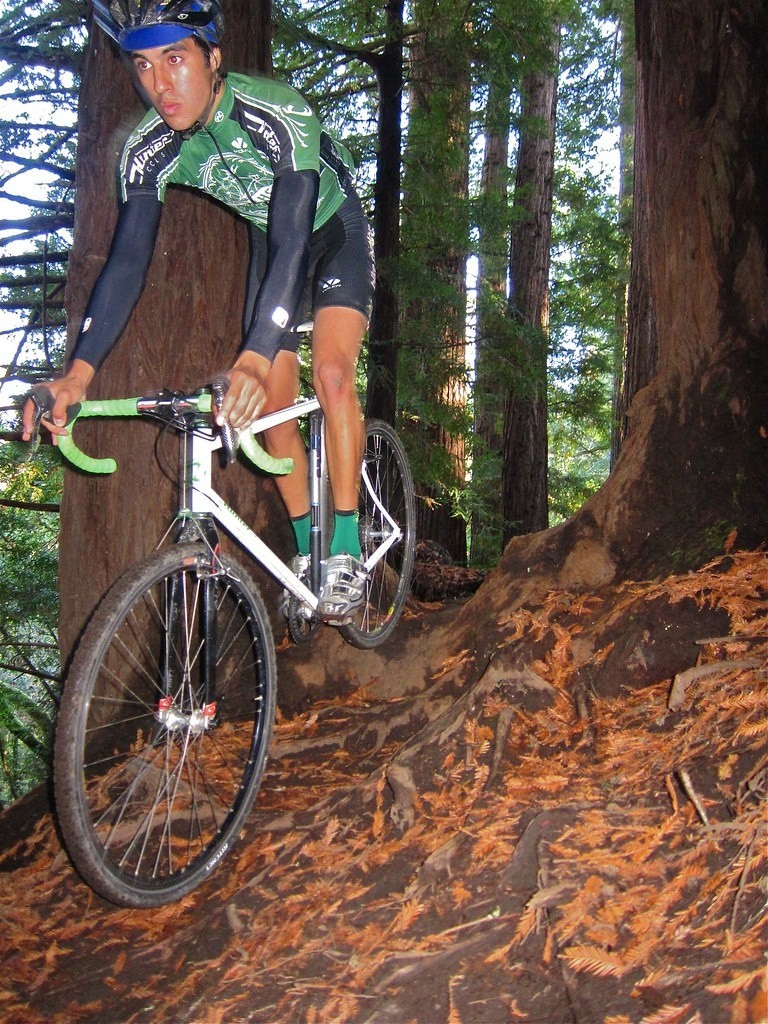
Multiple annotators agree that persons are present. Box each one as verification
[20,1,377,626]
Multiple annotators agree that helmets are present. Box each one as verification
[108,0,224,51]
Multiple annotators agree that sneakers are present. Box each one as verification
[283,552,370,627]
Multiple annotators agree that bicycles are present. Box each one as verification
[25,320,418,909]
